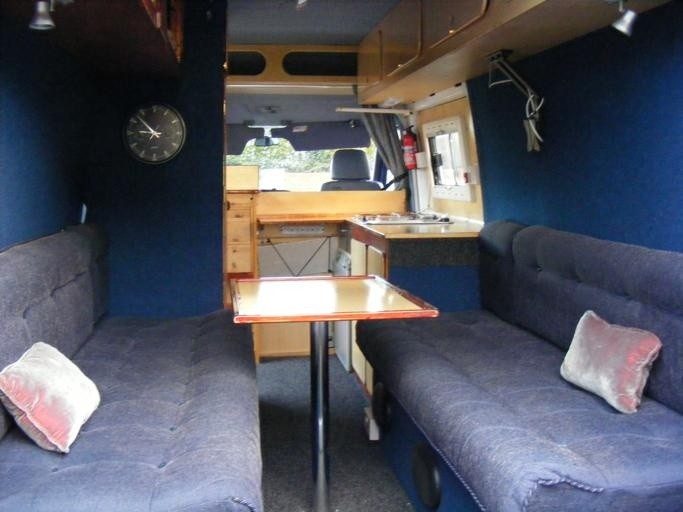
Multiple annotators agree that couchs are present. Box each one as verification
[0,220,259,511]
[354,218,682,512]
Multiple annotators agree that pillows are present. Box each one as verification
[1,342,100,454]
[557,308,663,414]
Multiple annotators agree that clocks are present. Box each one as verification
[123,102,186,166]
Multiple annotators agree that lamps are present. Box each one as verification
[606,0,637,36]
[29,1,56,29]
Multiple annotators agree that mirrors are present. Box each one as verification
[421,116,477,203]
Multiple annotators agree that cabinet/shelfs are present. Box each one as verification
[255,191,406,359]
[223,190,259,308]
[350,215,481,401]
[357,23,381,104]
[425,0,681,98]
[382,0,424,104]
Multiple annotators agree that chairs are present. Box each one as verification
[320,150,384,191]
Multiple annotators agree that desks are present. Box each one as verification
[230,275,439,510]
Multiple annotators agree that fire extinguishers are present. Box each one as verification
[402,125,418,171]
